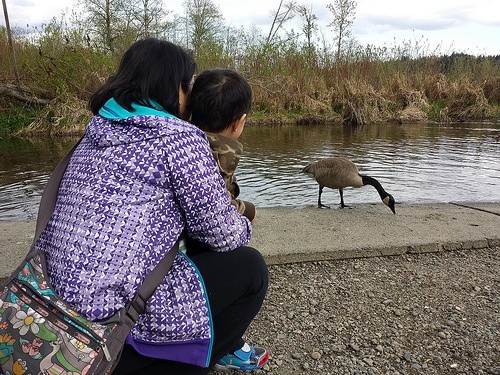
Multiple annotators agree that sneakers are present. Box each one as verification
[213,342,269,371]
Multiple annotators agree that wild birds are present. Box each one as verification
[298,156,396,215]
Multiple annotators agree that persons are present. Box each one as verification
[187,68,269,370]
[35,38,270,375]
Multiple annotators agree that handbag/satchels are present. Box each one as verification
[0,249,130,375]
